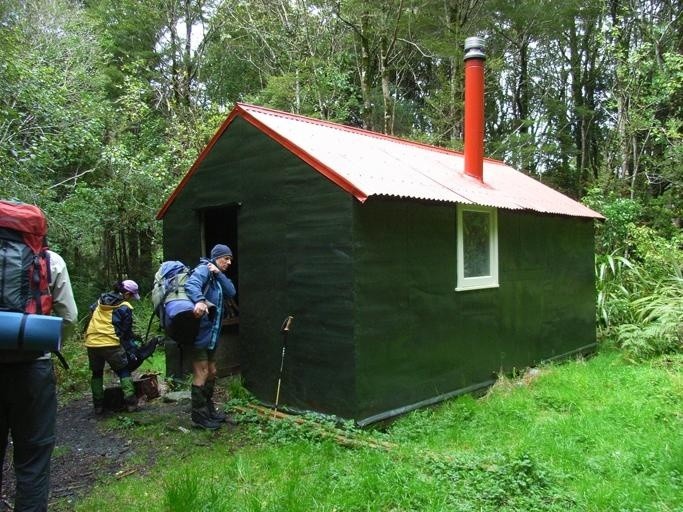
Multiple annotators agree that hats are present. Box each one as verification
[121,278,140,300]
[209,244,233,260]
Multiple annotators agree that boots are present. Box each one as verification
[119,376,145,413]
[190,382,221,430]
[90,376,105,415]
[204,379,229,423]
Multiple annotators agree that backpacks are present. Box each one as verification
[150,259,214,332]
[0,197,53,365]
[126,335,159,373]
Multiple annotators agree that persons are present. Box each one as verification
[178,245,237,430]
[0,242,78,512]
[84,279,147,417]
